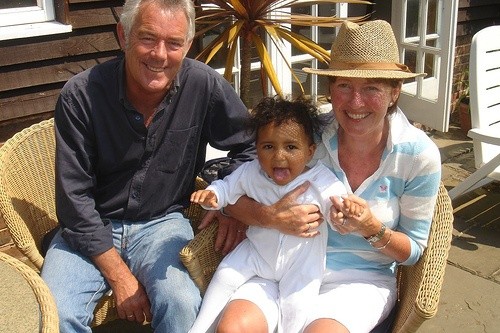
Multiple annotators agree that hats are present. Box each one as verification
[302,19,427,83]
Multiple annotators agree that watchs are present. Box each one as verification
[363,223,386,243]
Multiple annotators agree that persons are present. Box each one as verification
[216,19,442,333]
[39,0,258,333]
[188,92,363,333]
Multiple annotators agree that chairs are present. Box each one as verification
[0,117,210,329]
[179,179,454,333]
[431,26,500,199]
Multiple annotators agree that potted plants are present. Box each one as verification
[451,65,471,132]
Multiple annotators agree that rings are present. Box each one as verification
[306,224,310,231]
[342,219,346,225]
[237,230,244,233]
[127,314,135,318]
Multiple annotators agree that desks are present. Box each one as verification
[0,251,59,333]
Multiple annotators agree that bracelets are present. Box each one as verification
[376,229,392,250]
